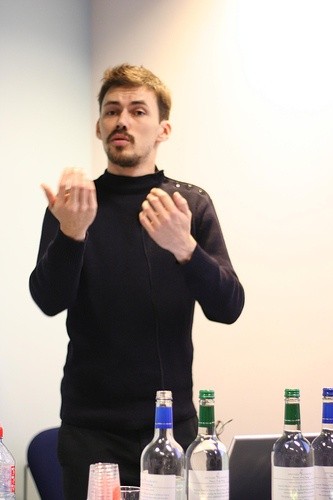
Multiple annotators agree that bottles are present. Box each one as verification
[311,388,333,500]
[139,390,183,500]
[270,388,314,500]
[0,427,16,500]
[184,390,231,500]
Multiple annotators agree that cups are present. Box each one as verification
[86,462,140,500]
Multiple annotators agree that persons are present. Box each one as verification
[27,64,245,500]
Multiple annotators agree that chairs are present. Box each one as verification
[23,428,65,500]
